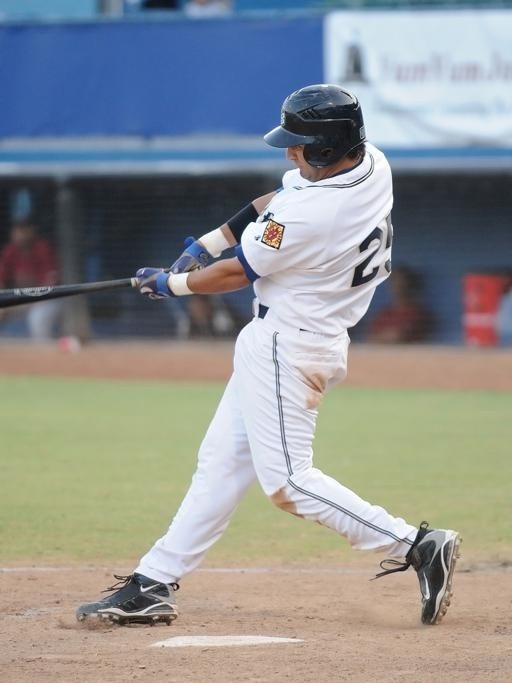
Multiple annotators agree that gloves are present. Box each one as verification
[137,267,176,301]
[168,240,214,274]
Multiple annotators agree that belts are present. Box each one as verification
[252,301,268,319]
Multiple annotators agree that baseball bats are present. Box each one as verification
[1,273,148,306]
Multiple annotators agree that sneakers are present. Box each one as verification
[76,570,178,626]
[407,522,462,625]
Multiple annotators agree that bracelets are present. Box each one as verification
[195,226,235,258]
[166,272,195,296]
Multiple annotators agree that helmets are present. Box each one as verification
[264,85,367,168]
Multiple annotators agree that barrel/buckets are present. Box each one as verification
[460,268,512,348]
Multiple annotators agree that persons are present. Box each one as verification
[171,290,249,343]
[463,259,505,352]
[485,261,511,350]
[74,80,465,637]
[1,204,60,340]
[359,263,433,344]
